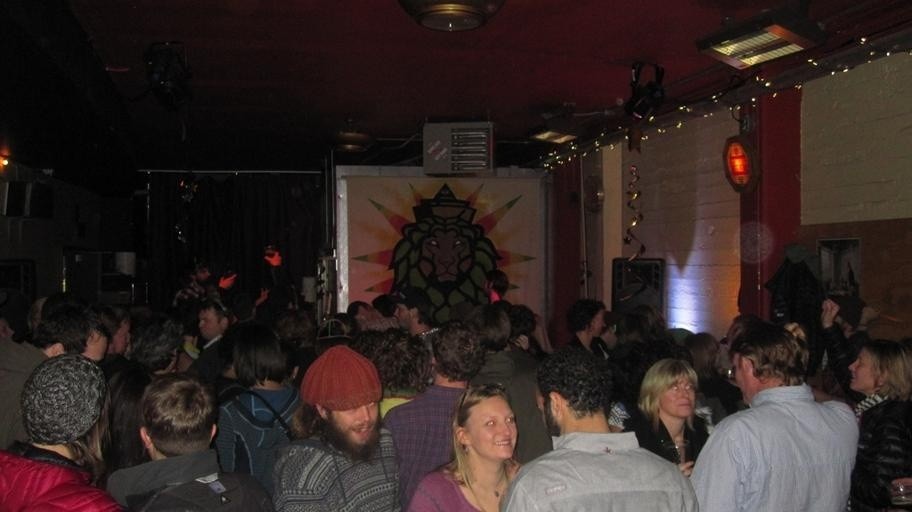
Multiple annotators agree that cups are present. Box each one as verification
[882,470,911,505]
[663,438,692,465]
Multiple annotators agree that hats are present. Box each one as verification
[300,344,383,411]
[389,286,431,315]
[19,353,108,445]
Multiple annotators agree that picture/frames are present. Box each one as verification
[335,165,554,332]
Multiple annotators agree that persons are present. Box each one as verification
[1,247,912,511]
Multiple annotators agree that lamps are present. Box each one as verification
[625,64,659,120]
[335,112,375,154]
[700,19,812,70]
[528,114,579,146]
[401,0,500,33]
